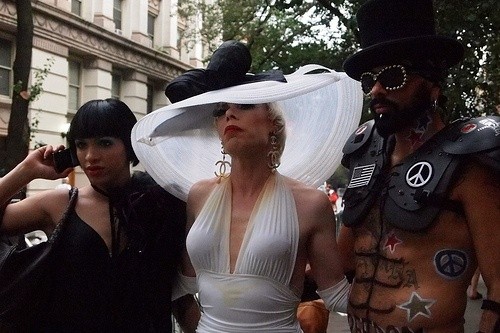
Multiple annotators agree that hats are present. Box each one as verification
[342,0,465,81]
[131,40,364,203]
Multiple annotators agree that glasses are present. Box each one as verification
[360,64,420,96]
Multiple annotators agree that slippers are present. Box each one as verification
[470,292,483,300]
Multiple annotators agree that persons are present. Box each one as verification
[0,97,200,333]
[299,3,500,333]
[129,39,365,332]
[326,185,339,212]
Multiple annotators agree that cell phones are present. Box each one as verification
[52,148,80,174]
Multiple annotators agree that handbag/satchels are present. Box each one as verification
[0,188,78,333]
